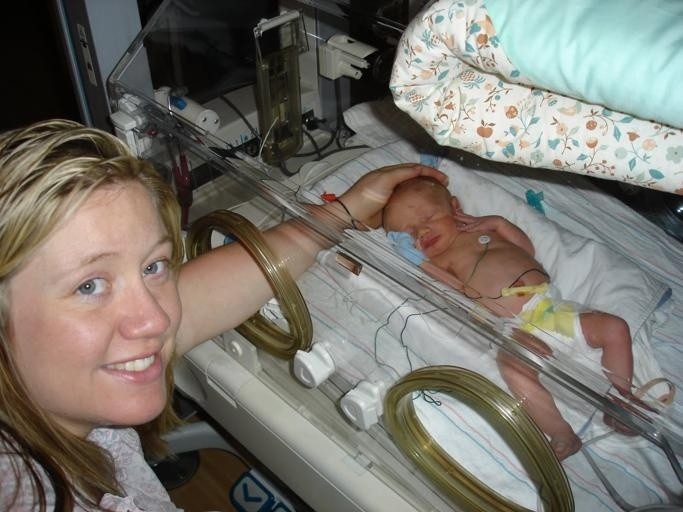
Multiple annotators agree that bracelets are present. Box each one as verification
[321,190,359,227]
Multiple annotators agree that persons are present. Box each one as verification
[1,118,449,512]
[382,175,658,462]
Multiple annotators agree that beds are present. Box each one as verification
[104,2,682,512]
